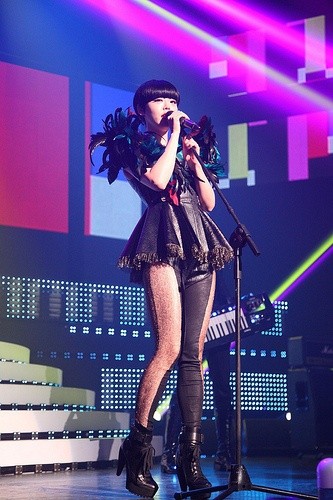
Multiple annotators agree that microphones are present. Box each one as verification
[165,111,201,131]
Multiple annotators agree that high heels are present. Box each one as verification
[175,433,212,500]
[117,423,159,498]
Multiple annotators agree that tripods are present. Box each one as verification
[174,124,319,500]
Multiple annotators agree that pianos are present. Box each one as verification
[203,289,276,353]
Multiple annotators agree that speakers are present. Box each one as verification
[284,368,333,453]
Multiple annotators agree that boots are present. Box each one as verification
[161,410,182,474]
[214,408,236,472]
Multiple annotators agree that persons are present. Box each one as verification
[84,79,237,500]
[160,253,243,475]
